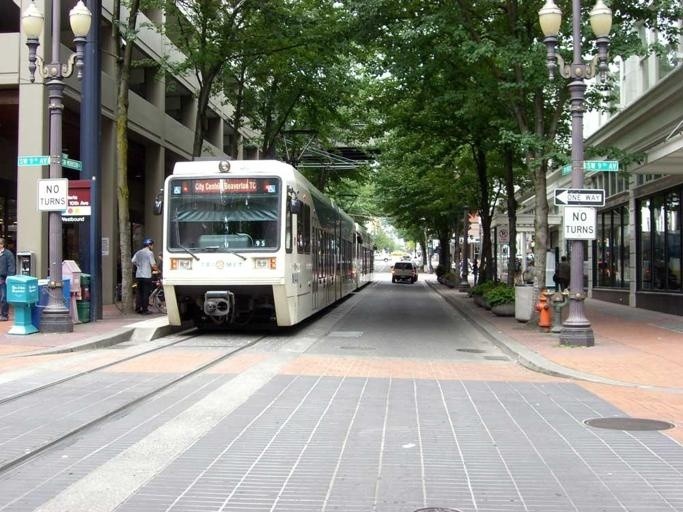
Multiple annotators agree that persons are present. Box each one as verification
[553,255,569,292]
[522,260,534,284]
[129,238,158,315]
[0,236,17,321]
[153,251,165,310]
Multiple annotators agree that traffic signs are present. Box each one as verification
[60,157,83,171]
[582,161,619,172]
[561,164,573,176]
[17,156,50,167]
[553,188,606,207]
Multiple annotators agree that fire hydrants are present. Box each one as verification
[535,293,554,328]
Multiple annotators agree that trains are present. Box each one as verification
[153,128,378,334]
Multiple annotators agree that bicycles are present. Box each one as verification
[114,269,167,315]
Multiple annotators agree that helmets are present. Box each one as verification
[144,239,154,244]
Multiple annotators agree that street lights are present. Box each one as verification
[19,1,93,334]
[536,0,613,348]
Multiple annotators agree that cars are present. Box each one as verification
[374,254,392,262]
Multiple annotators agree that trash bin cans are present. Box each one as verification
[514,284,534,320]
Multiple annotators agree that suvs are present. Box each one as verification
[390,261,418,284]
